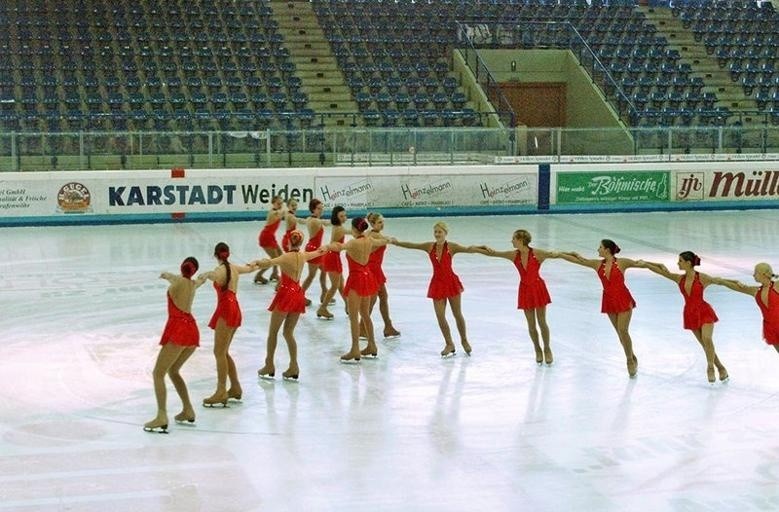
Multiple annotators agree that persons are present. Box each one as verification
[470,230,588,365]
[634,249,753,383]
[708,261,779,358]
[247,196,401,380]
[143,255,209,428]
[386,221,496,355]
[541,238,672,376]
[198,242,272,403]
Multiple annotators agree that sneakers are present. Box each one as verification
[537,350,553,363]
[627,358,637,375]
[306,294,335,317]
[384,329,400,335]
[258,366,299,378]
[145,412,195,429]
[441,340,472,355]
[708,368,727,382]
[255,273,280,283]
[341,347,376,360]
[203,389,241,404]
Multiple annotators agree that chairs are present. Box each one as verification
[317,0,779,155]
[1,0,316,153]
